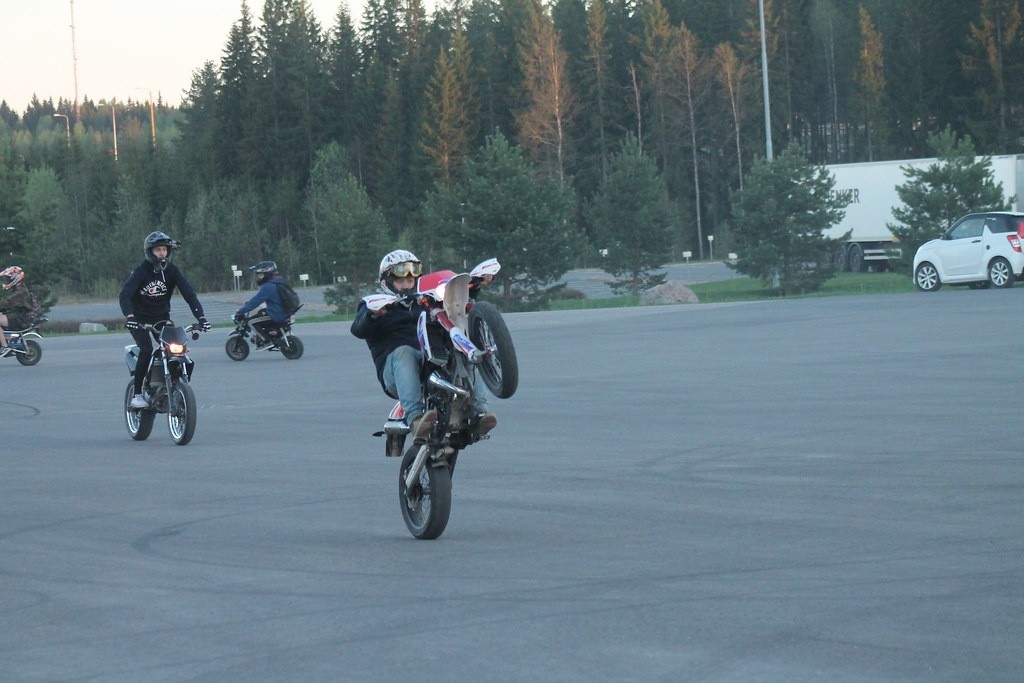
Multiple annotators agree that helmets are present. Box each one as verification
[0,266,25,290]
[144,232,175,267]
[379,250,420,278]
[250,262,277,285]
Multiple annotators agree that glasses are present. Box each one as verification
[386,261,423,278]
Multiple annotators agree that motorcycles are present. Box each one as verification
[0,319,48,366]
[225,303,305,361]
[124,320,210,445]
[371,258,519,543]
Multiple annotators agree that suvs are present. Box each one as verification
[912,211,1024,292]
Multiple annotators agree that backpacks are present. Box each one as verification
[275,282,300,313]
[26,290,41,324]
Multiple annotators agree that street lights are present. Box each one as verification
[98,104,117,160]
[135,87,156,146]
[53,114,70,145]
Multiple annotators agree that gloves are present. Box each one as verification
[127,316,138,331]
[199,317,211,332]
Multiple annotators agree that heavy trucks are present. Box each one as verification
[789,153,1024,272]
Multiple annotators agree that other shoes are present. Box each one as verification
[0,346,12,357]
[411,411,435,440]
[130,393,149,408]
[255,343,275,352]
[470,414,496,437]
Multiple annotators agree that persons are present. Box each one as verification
[235,261,291,352]
[350,250,498,445]
[118,231,211,408]
[0,266,33,357]
